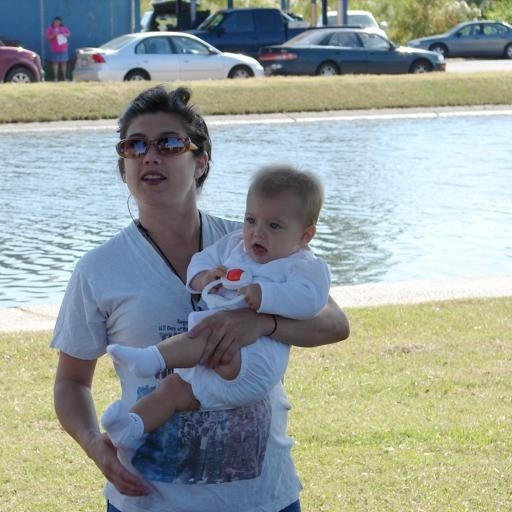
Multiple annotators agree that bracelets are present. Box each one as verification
[265,315,277,336]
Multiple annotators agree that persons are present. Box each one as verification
[101,164,330,449]
[46,16,71,82]
[51,84,350,512]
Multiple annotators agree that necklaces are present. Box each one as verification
[138,212,202,311]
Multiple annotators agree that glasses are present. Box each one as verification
[116,134,200,159]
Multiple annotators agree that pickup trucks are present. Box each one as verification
[181,5,363,62]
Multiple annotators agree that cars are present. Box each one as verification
[258,24,447,77]
[71,31,265,83]
[0,39,46,85]
[404,18,511,61]
[314,10,389,43]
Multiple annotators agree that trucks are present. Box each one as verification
[140,2,307,35]
